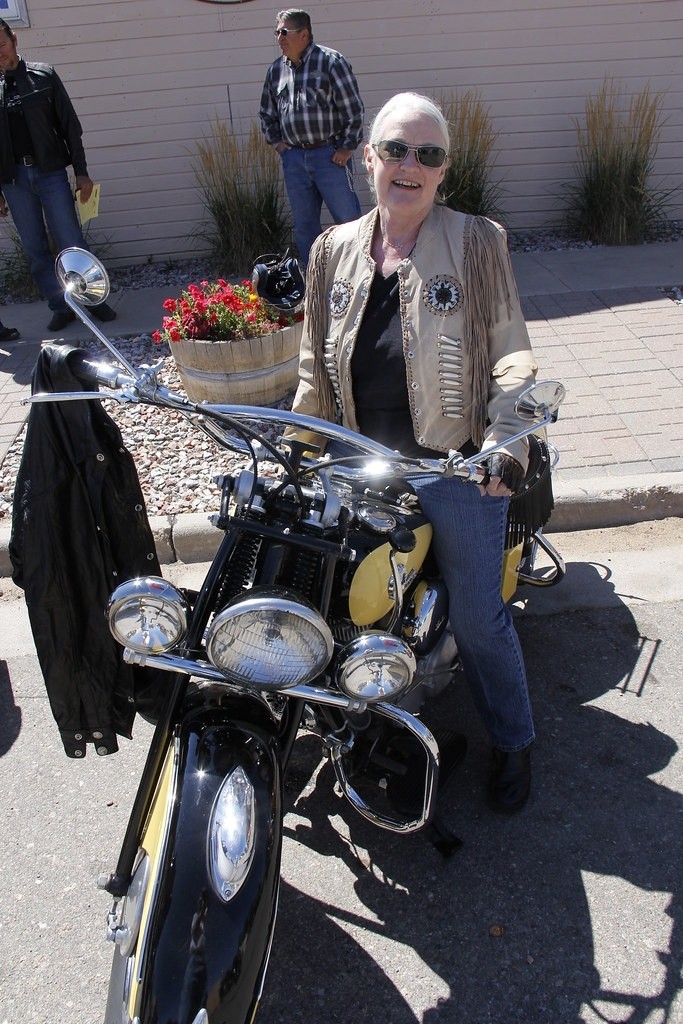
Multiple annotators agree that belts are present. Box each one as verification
[285,136,334,149]
[15,155,37,166]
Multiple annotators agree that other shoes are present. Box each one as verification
[47,312,75,331]
[0,321,20,340]
[492,746,531,813]
[84,301,116,320]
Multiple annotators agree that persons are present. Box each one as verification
[260,8,364,266]
[283,92,555,818]
[0,19,118,341]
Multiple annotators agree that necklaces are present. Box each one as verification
[376,223,418,251]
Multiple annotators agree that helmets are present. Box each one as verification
[252,247,305,314]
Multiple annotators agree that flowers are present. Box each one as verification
[152,279,304,344]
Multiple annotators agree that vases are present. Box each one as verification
[165,320,303,405]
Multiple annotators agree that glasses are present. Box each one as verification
[274,28,298,36]
[371,139,448,169]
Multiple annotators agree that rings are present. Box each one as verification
[339,161,342,163]
